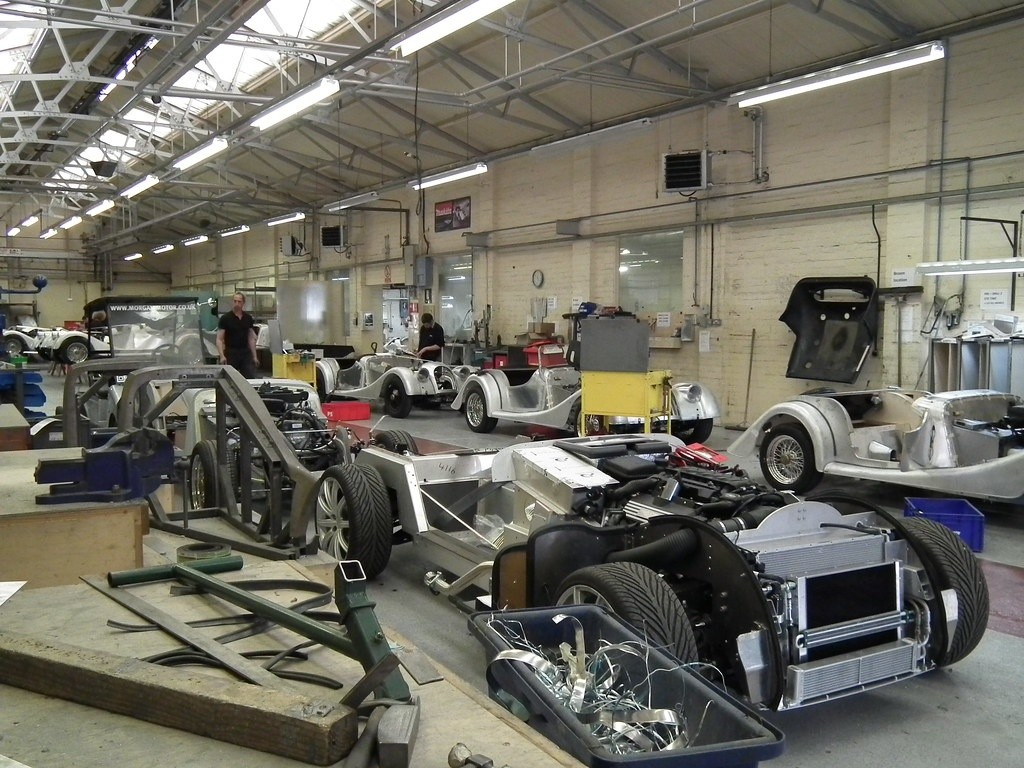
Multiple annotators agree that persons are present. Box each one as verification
[215,291,261,379]
[416,312,445,362]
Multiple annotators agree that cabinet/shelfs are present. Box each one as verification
[440,344,472,377]
[0,447,150,593]
[580,318,672,437]
[273,354,317,389]
[0,402,30,451]
[0,560,589,768]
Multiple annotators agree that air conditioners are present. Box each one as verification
[281,235,302,256]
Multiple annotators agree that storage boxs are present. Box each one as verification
[27,411,91,449]
[475,322,568,370]
[468,603,786,768]
[905,497,985,552]
[321,401,371,422]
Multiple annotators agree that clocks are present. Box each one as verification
[533,270,543,287]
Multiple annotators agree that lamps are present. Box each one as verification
[172,138,228,170]
[408,163,488,190]
[531,118,654,156]
[323,191,379,212]
[249,76,340,131]
[916,257,1024,276]
[388,0,515,57]
[85,199,115,216]
[119,174,159,198]
[728,41,944,109]
[7,212,306,260]
